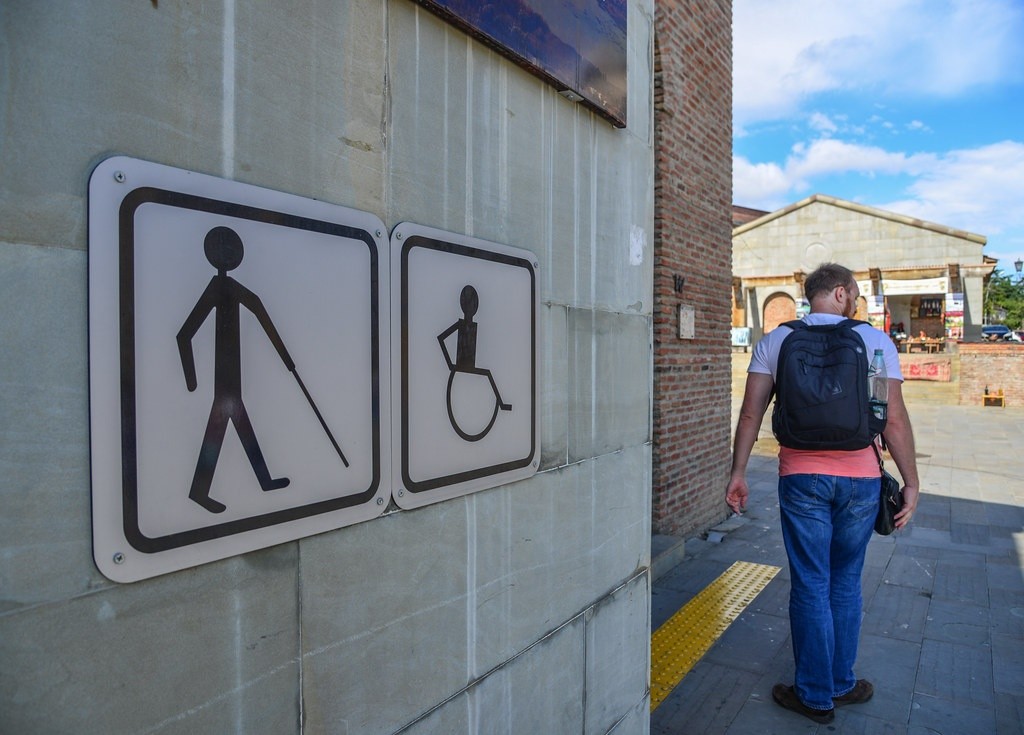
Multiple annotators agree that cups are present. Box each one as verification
[999,390,1003,396]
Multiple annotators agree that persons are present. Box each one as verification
[725,264,919,724]
[890,317,904,351]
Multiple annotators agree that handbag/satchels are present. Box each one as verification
[873,469,905,535]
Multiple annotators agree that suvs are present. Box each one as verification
[982,324,1009,339]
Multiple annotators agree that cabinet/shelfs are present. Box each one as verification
[918,298,942,318]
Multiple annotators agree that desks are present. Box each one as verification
[899,340,945,353]
[982,395,1005,407]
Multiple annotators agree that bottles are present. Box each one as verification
[984,385,988,395]
[868,349,889,419]
[921,300,941,314]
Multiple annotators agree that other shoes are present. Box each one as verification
[832,678,874,706]
[772,682,836,724]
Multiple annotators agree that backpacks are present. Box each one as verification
[756,318,888,452]
